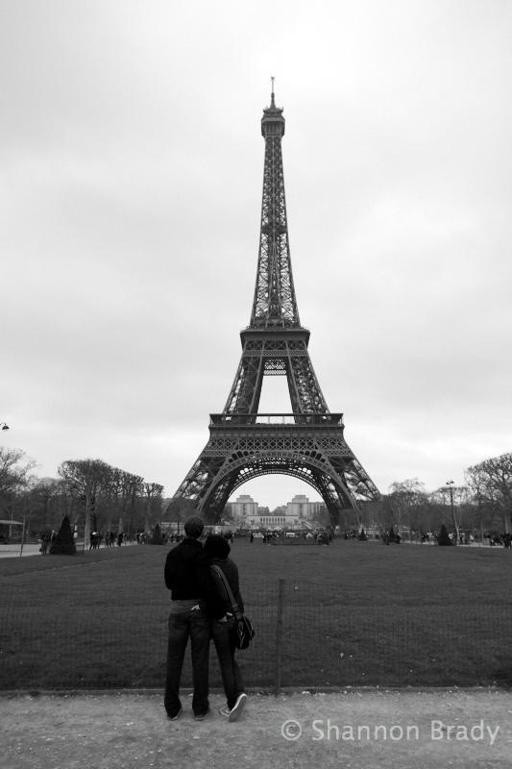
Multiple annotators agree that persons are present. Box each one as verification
[90,523,511,564]
[190,535,248,723]
[39,528,56,556]
[164,517,233,721]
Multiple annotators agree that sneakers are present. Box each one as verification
[194,708,209,720]
[220,692,248,722]
[169,704,183,720]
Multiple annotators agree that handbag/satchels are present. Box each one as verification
[234,616,256,649]
